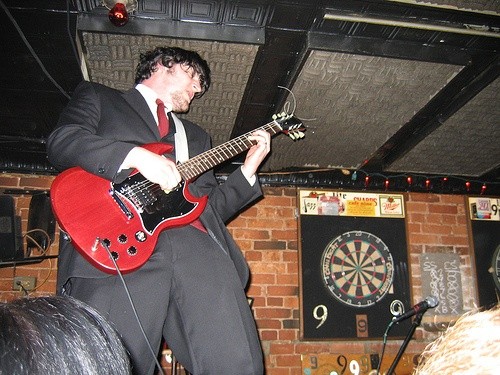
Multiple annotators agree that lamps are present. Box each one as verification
[102,0,138,25]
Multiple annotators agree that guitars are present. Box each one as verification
[47,111,307,276]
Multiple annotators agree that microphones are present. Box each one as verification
[392,295,440,323]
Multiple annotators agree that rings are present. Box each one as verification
[263,142,267,145]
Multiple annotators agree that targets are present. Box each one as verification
[320,230,394,307]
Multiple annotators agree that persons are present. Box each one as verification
[52,44,272,375]
[0,291,133,374]
[412,296,500,375]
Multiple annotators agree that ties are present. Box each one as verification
[155,99,170,139]
